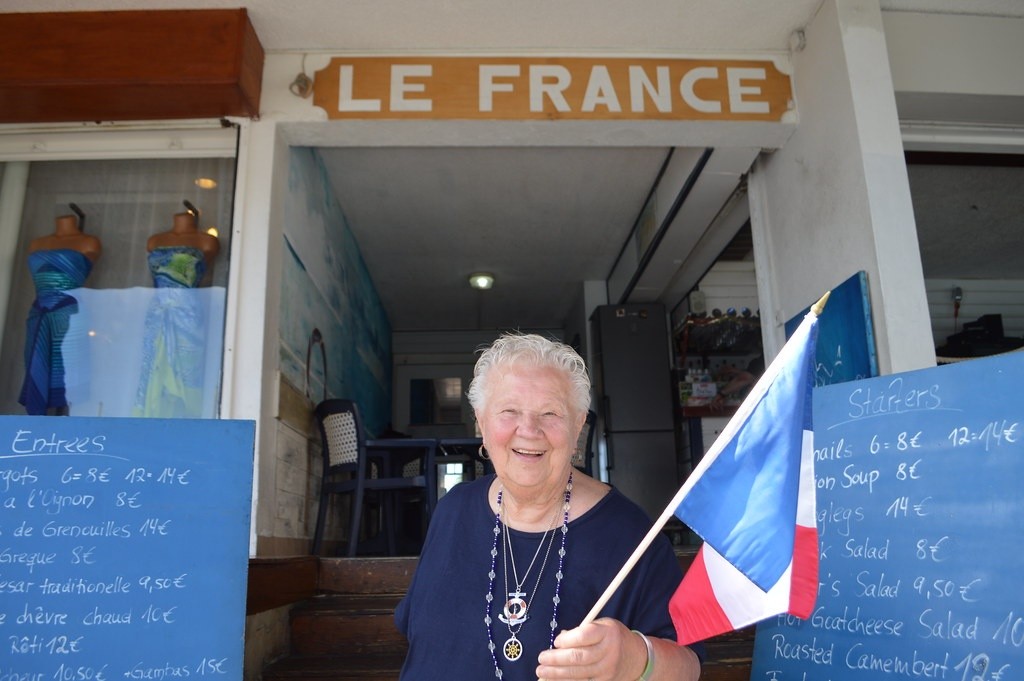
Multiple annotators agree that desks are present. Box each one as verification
[363,434,485,554]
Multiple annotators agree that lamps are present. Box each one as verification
[469,273,494,289]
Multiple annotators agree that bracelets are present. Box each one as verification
[632,630,654,681]
[717,391,725,399]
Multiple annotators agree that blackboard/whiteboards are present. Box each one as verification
[751,349,1023,681]
[0,414,256,681]
[783,268,880,388]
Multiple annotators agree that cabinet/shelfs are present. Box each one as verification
[671,315,763,490]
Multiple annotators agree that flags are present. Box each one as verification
[669,317,819,646]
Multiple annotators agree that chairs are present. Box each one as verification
[310,398,438,557]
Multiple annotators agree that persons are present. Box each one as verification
[17,216,102,416]
[394,335,705,681]
[709,327,765,414]
[134,214,219,420]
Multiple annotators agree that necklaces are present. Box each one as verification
[484,472,573,681]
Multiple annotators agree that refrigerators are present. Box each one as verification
[588,305,682,543]
